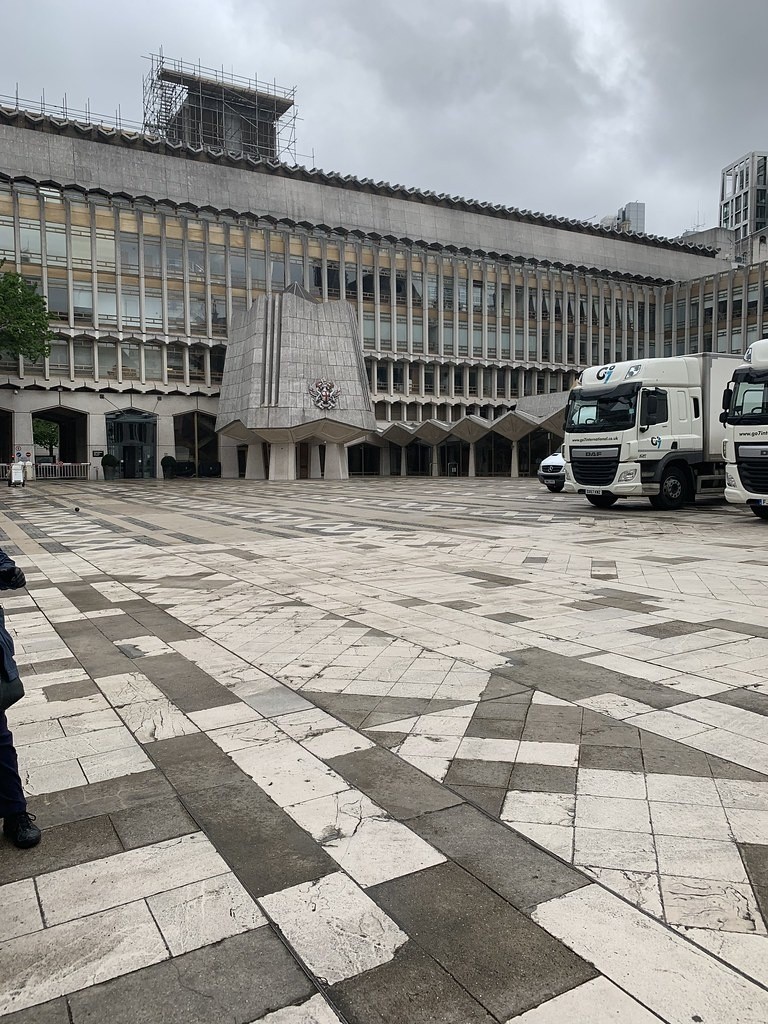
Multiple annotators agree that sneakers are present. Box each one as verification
[3,812,41,849]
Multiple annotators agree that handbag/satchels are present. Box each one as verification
[0,662,25,711]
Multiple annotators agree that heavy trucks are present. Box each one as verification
[719,339,768,522]
[563,353,751,513]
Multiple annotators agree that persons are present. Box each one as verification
[0,550,41,847]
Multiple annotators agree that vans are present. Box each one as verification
[538,444,567,492]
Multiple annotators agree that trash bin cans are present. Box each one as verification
[8,462,25,486]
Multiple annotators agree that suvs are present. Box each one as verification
[35,455,55,474]
